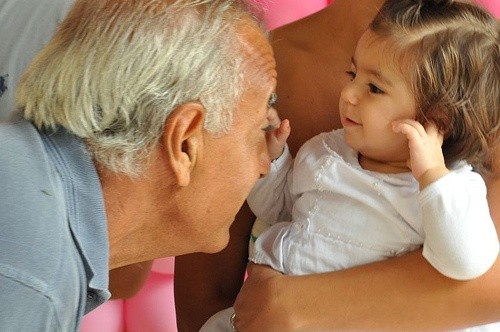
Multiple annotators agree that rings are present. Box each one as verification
[230,313,237,328]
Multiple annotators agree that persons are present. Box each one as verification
[198,0,500,332]
[0,0,277,332]
[173,0,500,332]
